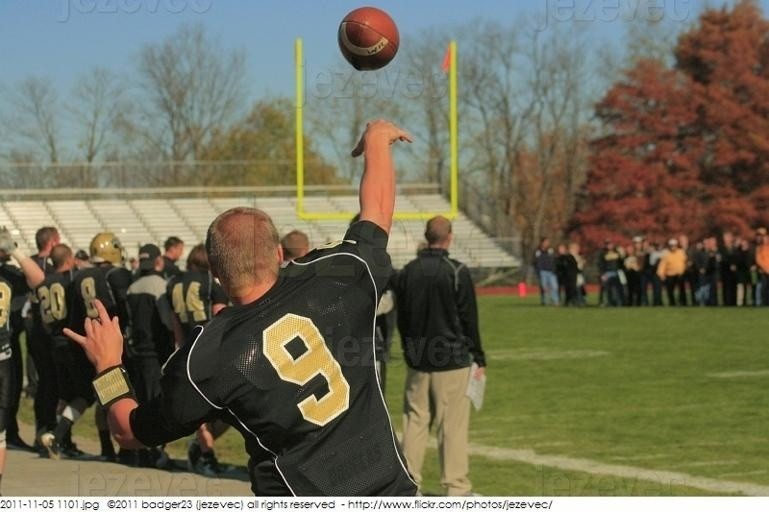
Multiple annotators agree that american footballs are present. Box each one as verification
[338,8,399,70]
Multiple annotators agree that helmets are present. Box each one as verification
[89,232,124,265]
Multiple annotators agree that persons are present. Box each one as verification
[166,244,228,476]
[0,223,139,496]
[282,228,311,267]
[596,227,769,308]
[64,118,415,496]
[534,235,589,307]
[396,214,486,496]
[374,267,401,392]
[126,244,186,470]
[162,236,184,274]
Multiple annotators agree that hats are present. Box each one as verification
[139,244,161,272]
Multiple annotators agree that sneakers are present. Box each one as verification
[60,439,84,458]
[97,441,219,479]
[6,437,36,451]
[41,430,62,462]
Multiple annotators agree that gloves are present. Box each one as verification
[0,225,18,257]
